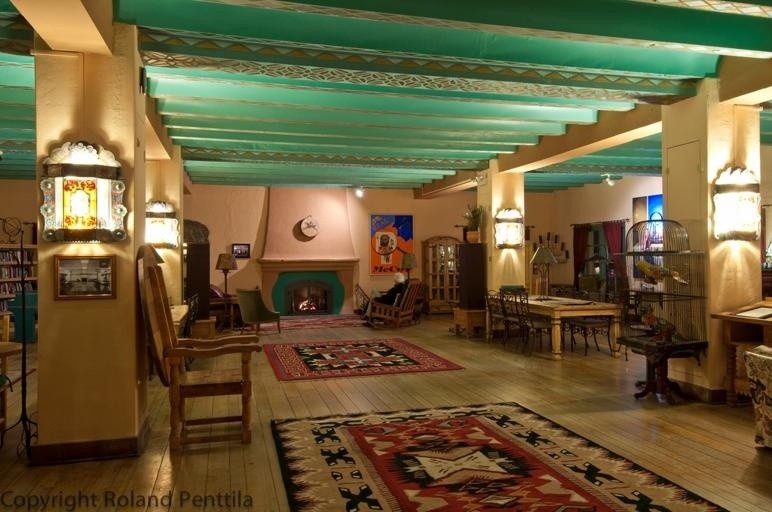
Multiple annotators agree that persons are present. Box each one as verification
[353,272,406,328]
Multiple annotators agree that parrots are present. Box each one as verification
[642,313,689,344]
[636,260,689,285]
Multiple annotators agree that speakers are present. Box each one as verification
[186,242,210,319]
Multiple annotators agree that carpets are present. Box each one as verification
[263,337,465,383]
[255,313,366,330]
[268,401,728,512]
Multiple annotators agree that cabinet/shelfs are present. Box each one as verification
[0,241,37,316]
[423,236,463,313]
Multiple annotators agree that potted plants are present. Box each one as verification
[460,200,484,243]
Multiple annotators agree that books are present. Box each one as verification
[0,250,33,312]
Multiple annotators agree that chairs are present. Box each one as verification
[742,345,772,451]
[370,280,420,328]
[146,265,282,454]
[486,289,640,363]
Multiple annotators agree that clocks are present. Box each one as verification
[299,215,322,238]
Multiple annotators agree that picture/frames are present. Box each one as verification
[232,243,251,258]
[52,253,119,303]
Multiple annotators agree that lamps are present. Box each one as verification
[528,245,559,300]
[215,254,239,297]
[400,253,417,279]
[581,242,617,279]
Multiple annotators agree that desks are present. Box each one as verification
[710,302,772,412]
[616,335,708,406]
[451,308,486,342]
